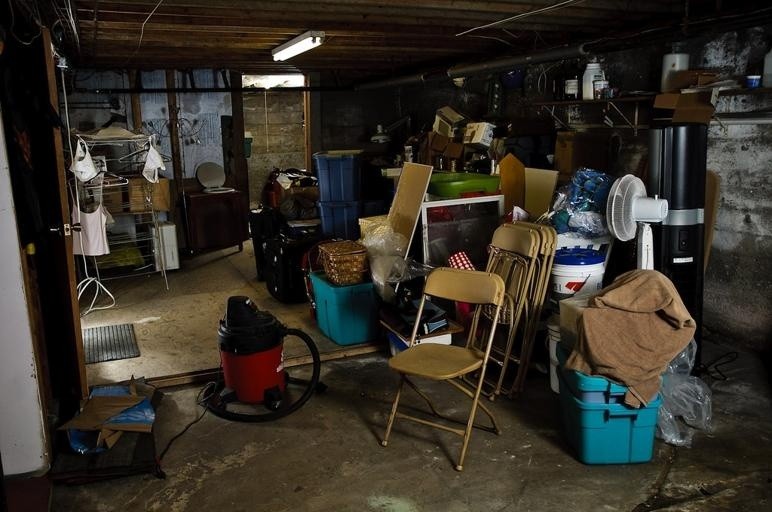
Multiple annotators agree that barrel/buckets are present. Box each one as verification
[550,248,605,323]
[545,318,562,395]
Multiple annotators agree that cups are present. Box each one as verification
[745,75,761,88]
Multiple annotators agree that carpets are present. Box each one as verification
[81,324,141,364]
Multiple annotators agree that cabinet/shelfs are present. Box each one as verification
[533,94,656,136]
[654,86,772,125]
[77,183,170,296]
[182,191,249,256]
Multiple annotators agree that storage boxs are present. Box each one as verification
[312,149,370,241]
[245,131,253,158]
[126,175,171,212]
[93,180,123,214]
[653,70,722,123]
[555,341,665,466]
[414,106,502,197]
[308,269,377,346]
[554,131,592,174]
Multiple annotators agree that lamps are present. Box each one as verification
[271,30,326,62]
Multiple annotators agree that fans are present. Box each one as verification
[605,174,668,271]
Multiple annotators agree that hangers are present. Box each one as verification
[67,159,129,189]
[118,136,174,164]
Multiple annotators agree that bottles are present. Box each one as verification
[564,63,609,99]
